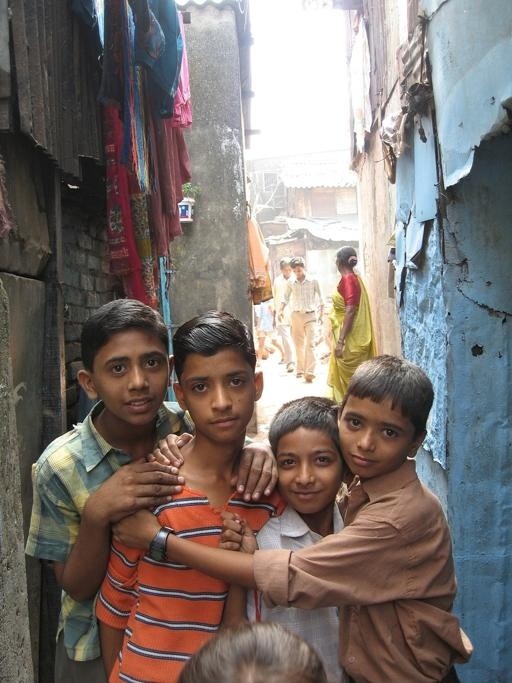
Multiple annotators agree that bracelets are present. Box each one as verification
[337,338,344,344]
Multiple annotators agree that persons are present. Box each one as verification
[277,255,326,383]
[173,619,329,681]
[272,258,298,373]
[95,309,287,681]
[111,350,473,682]
[330,242,377,404]
[23,296,282,681]
[219,393,359,680]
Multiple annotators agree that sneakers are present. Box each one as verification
[278,358,317,384]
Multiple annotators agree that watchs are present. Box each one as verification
[150,524,175,562]
